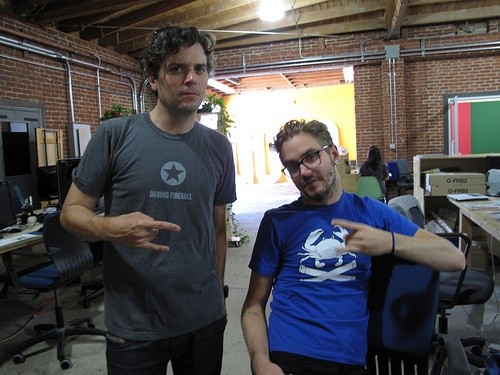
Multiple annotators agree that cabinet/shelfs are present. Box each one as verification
[412,153,500,264]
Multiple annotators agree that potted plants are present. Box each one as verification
[99,103,131,121]
[202,92,235,139]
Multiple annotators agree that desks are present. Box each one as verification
[446,191,500,268]
[0,197,110,264]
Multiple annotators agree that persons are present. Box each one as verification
[359,146,389,196]
[241,120,466,375]
[59,26,236,375]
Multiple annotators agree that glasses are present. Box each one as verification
[281,145,329,178]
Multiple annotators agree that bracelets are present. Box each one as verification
[391,232,395,255]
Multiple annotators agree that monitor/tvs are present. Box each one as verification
[56,158,82,210]
[4,174,42,213]
[0,181,18,230]
[34,165,59,201]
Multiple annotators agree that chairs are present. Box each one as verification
[386,162,414,196]
[355,175,387,207]
[389,194,495,354]
[395,158,410,180]
[9,207,109,371]
[357,253,474,375]
